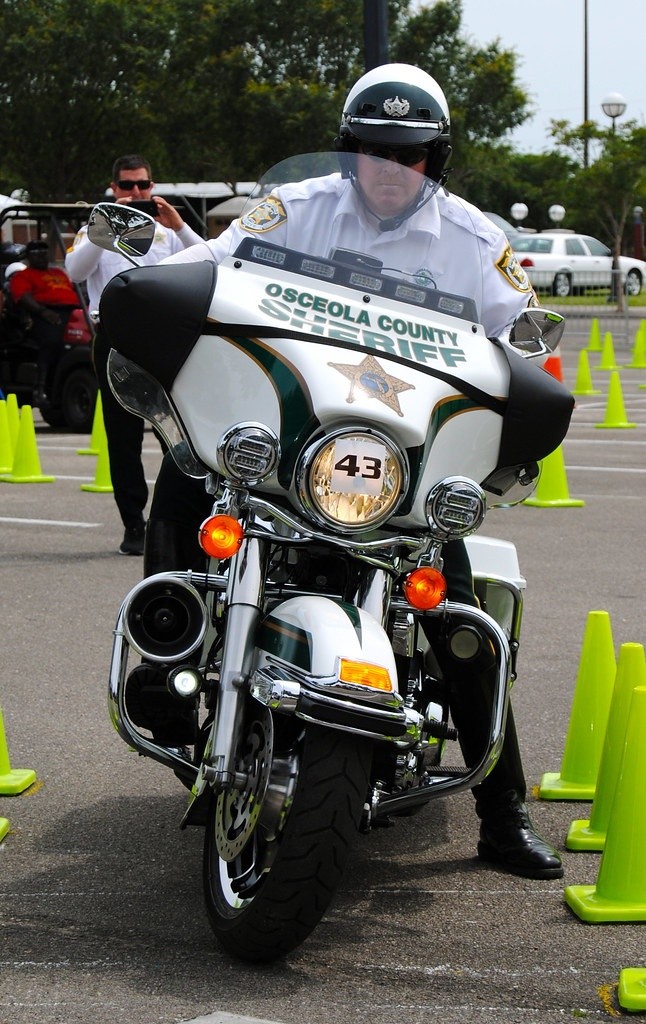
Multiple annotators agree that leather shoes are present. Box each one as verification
[474,802,562,880]
[120,663,196,755]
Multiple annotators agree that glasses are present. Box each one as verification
[361,146,429,167]
[117,180,153,189]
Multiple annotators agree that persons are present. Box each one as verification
[12,241,91,342]
[127,62,568,880]
[65,156,205,554]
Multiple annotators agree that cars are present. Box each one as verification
[510,233,646,297]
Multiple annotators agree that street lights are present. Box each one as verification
[601,92,627,170]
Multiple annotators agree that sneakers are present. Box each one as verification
[120,525,146,555]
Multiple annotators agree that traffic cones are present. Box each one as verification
[82,428,114,493]
[77,389,109,455]
[593,331,623,370]
[618,966,646,1014]
[624,318,646,368]
[595,370,635,428]
[0,709,38,845]
[539,611,646,922]
[586,317,602,352]
[569,350,601,394]
[0,393,55,483]
[525,439,586,508]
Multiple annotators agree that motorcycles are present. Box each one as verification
[86,151,566,966]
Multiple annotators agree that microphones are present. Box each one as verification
[380,175,448,232]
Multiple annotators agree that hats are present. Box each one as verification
[26,240,50,253]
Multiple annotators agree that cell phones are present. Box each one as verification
[127,200,158,217]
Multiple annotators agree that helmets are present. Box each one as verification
[334,62,454,187]
